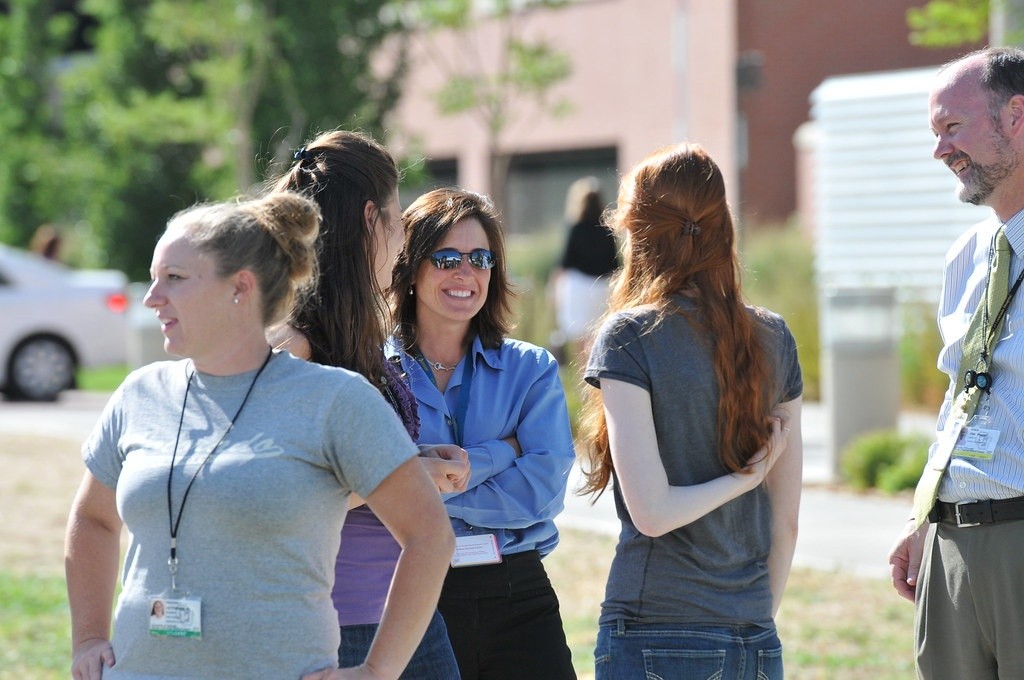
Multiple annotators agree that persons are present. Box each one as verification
[955,427,968,444]
[381,187,578,678]
[268,131,463,678]
[150,601,166,627]
[553,181,620,369]
[64,193,456,679]
[886,44,1024,680]
[30,226,62,262]
[583,148,803,680]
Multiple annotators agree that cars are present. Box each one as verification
[1,243,135,403]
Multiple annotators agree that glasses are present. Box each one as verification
[424,250,499,270]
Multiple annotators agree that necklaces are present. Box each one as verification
[425,358,458,372]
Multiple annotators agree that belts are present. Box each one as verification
[933,496,1024,526]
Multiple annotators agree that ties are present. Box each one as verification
[914,233,1010,530]
